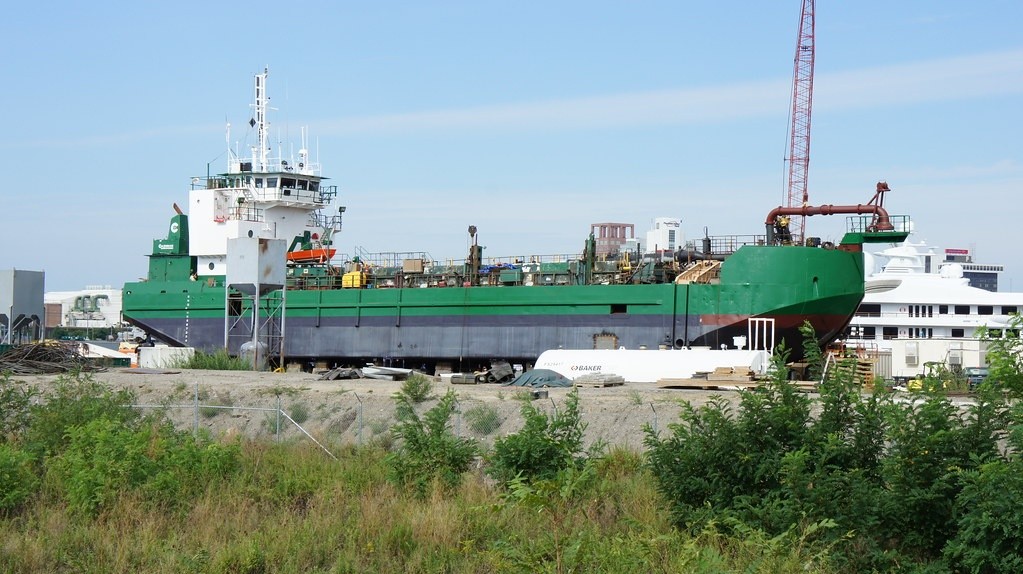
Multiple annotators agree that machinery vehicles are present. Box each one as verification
[782,0,818,247]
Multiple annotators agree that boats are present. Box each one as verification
[120,60,908,368]
[833,219,1023,389]
